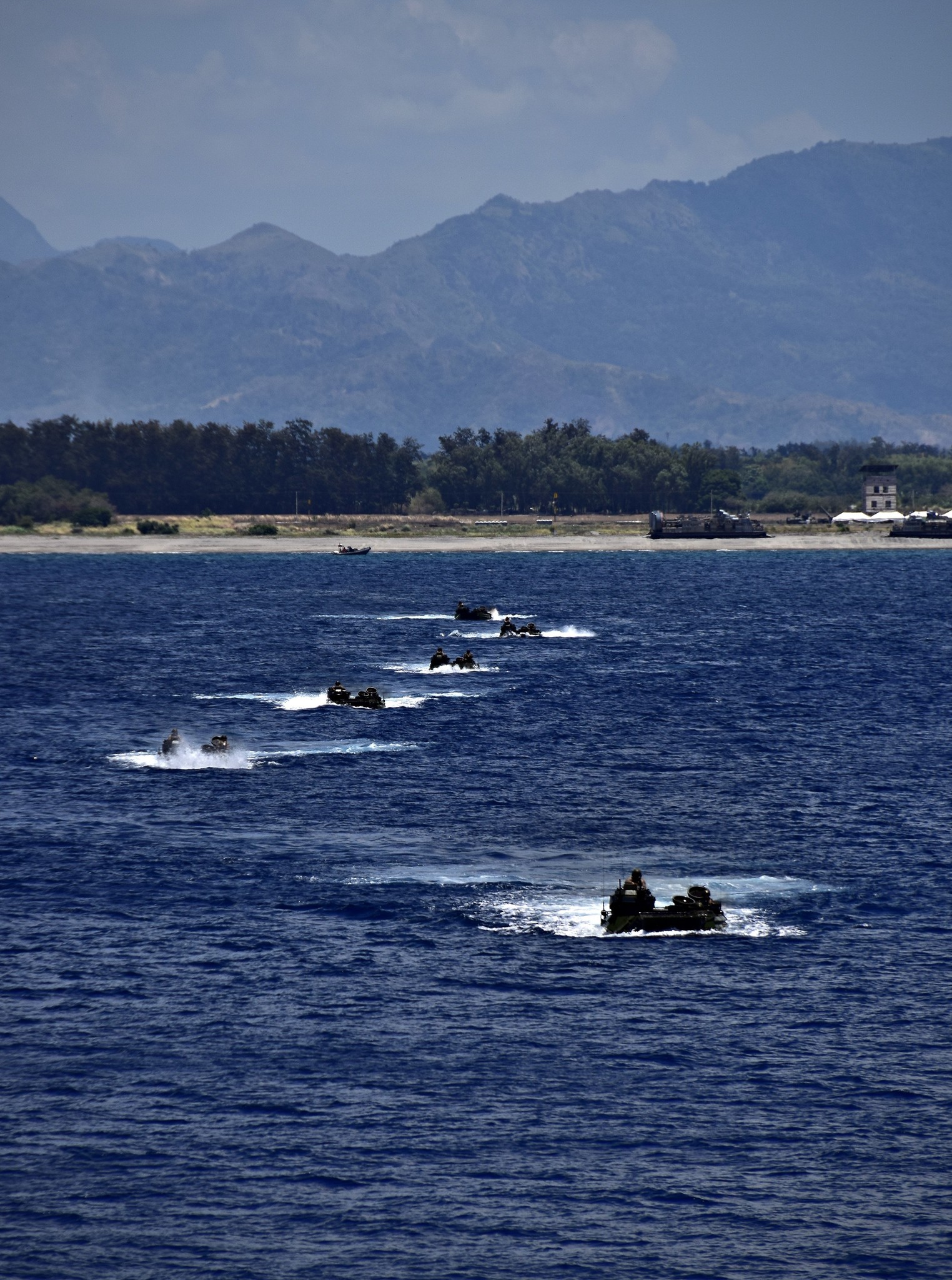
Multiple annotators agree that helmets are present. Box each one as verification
[632,869,642,878]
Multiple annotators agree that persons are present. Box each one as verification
[168,728,181,741]
[435,647,444,657]
[624,870,646,890]
[458,601,463,607]
[464,650,472,659]
[332,681,344,690]
[215,735,227,748]
[504,617,510,625]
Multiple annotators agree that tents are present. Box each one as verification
[831,511,870,525]
[905,511,939,522]
[871,511,905,524]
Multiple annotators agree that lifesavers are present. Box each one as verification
[456,657,464,661]
[527,623,535,628]
[358,691,369,697]
[365,687,377,694]
[479,606,485,610]
[521,626,528,631]
[462,653,473,658]
[210,736,227,746]
[201,744,217,752]
[672,895,696,906]
[473,609,480,612]
[688,886,710,900]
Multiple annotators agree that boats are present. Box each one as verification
[599,879,727,935]
[156,737,181,759]
[454,605,492,620]
[327,683,386,710]
[429,653,479,670]
[333,544,371,555]
[201,735,231,756]
[499,623,543,638]
